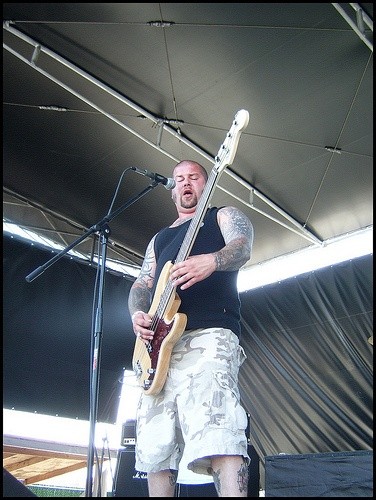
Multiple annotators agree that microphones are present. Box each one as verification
[132,166,176,192]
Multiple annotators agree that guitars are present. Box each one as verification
[132,109,249,396]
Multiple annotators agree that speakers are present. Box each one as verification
[113,448,178,498]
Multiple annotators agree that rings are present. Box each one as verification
[137,332,142,338]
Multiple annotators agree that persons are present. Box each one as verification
[126,159,255,498]
